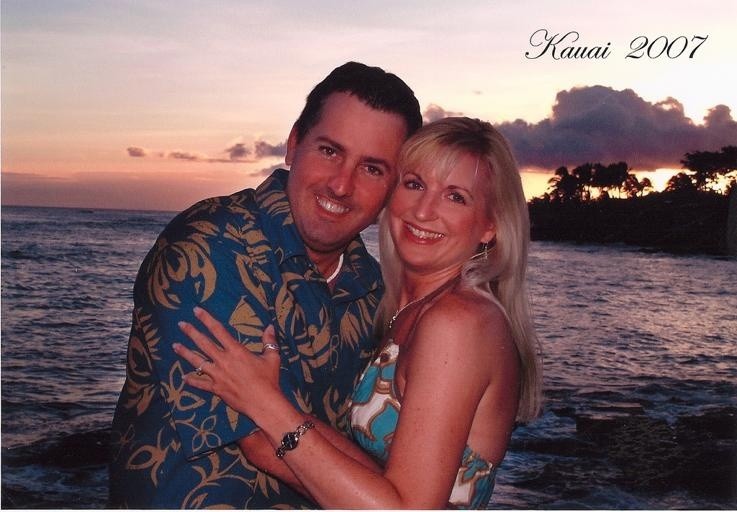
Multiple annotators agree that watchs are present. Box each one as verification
[268,417,315,460]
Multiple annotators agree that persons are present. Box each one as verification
[105,58,425,510]
[168,113,548,509]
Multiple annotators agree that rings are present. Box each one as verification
[261,342,279,352]
[192,361,207,377]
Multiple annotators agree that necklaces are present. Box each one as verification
[385,295,423,331]
[316,251,348,286]
